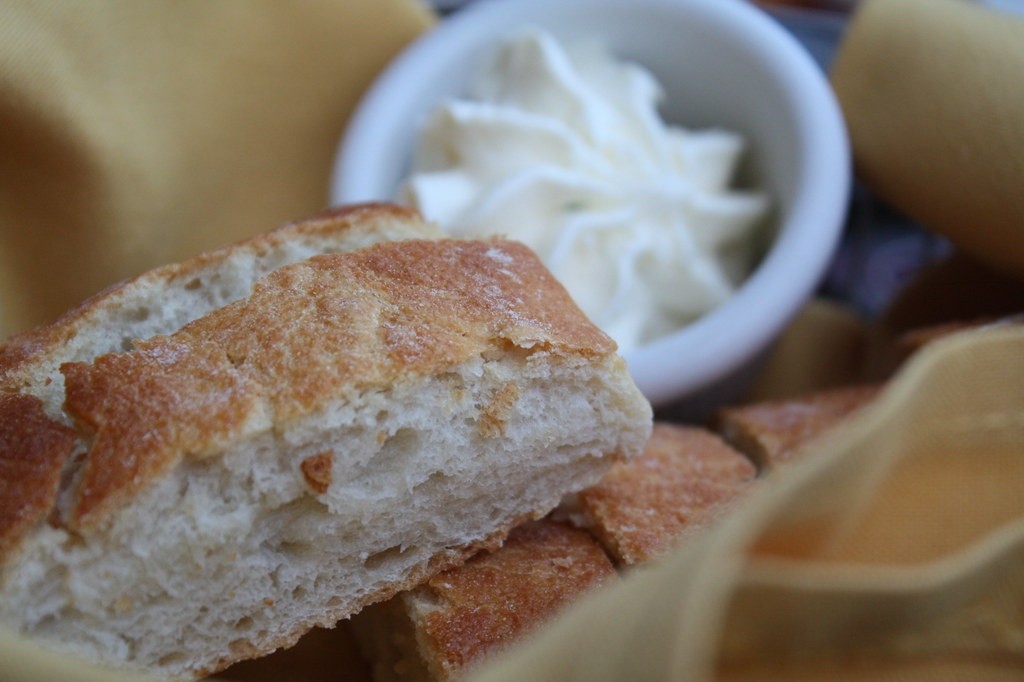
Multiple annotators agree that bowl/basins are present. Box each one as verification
[328,0,849,408]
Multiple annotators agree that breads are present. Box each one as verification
[0,202,994,682]
